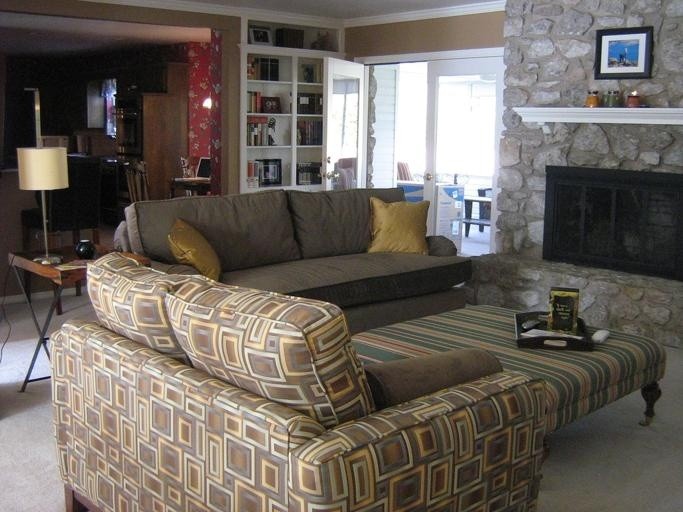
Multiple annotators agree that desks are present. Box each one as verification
[166,176,209,200]
[7,247,147,393]
[462,196,491,238]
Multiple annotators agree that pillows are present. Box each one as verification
[361,194,432,258]
[164,214,223,283]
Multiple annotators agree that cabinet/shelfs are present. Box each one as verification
[236,17,346,193]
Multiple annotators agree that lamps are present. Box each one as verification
[14,145,70,266]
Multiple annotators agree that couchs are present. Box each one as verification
[48,250,548,511]
[111,185,476,337]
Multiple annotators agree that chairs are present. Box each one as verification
[19,153,99,318]
[336,167,354,190]
[123,167,152,202]
[332,157,356,181]
[396,162,413,182]
[477,188,492,232]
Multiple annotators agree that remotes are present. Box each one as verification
[522,318,543,331]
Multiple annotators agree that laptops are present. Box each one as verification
[174,156,212,182]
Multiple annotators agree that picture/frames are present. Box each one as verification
[591,26,655,81]
[254,159,283,185]
[260,96,281,114]
[248,27,273,47]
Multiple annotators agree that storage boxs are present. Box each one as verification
[396,182,463,254]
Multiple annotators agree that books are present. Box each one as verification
[53,259,97,271]
[246,159,282,188]
[246,55,279,81]
[297,92,322,114]
[297,63,321,83]
[520,329,584,347]
[275,28,304,49]
[246,117,270,146]
[246,91,264,113]
[296,119,321,145]
[296,161,321,185]
